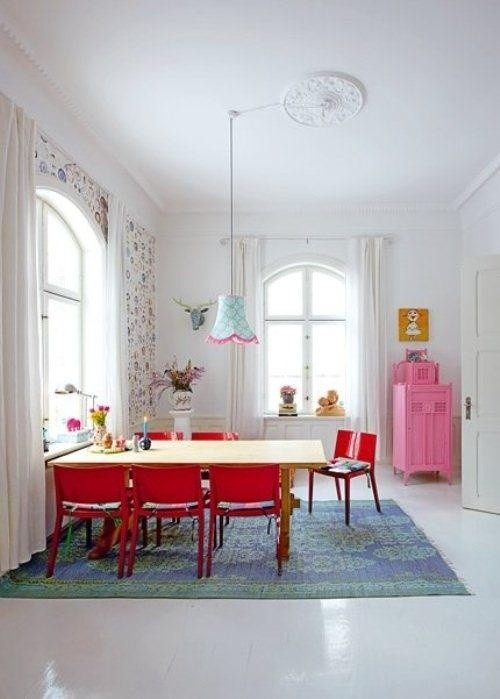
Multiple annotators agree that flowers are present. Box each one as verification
[90,406,110,426]
[280,386,296,396]
[150,355,207,401]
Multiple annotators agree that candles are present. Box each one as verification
[143,420,148,437]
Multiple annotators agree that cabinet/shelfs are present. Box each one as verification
[390,349,453,486]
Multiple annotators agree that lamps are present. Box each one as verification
[54,384,96,408]
[205,110,259,346]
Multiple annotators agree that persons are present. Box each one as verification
[317,390,345,417]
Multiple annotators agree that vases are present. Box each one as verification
[283,396,294,404]
[173,390,193,410]
[92,427,107,447]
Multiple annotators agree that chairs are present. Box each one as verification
[45,464,148,578]
[127,465,209,579]
[207,463,283,578]
[308,429,381,524]
[135,431,240,440]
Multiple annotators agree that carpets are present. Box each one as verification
[1,500,477,599]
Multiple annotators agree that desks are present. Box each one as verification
[46,439,328,560]
